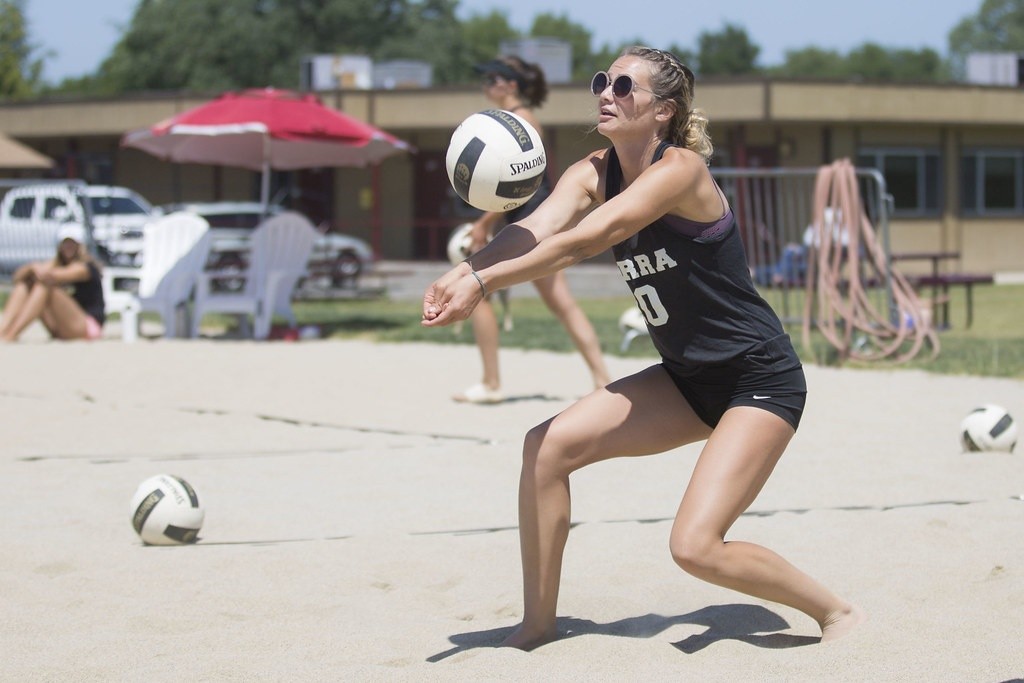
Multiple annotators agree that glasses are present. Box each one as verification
[590,70,666,103]
[490,79,497,86]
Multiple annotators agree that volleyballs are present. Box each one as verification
[960,401,1021,455]
[447,223,495,265]
[132,474,207,547]
[446,106,548,214]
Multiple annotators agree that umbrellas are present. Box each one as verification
[124,90,414,223]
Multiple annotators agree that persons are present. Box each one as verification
[421,48,867,650]
[749,185,851,285]
[454,54,612,403]
[0,221,108,343]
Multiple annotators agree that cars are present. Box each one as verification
[183,201,373,293]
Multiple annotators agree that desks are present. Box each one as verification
[809,250,961,327]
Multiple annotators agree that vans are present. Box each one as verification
[0,185,166,289]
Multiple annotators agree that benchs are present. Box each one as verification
[774,273,993,327]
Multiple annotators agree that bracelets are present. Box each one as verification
[472,271,485,297]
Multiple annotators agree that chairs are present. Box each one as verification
[188,210,323,340]
[104,211,211,344]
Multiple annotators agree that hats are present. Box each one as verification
[471,57,528,91]
[56,222,86,250]
